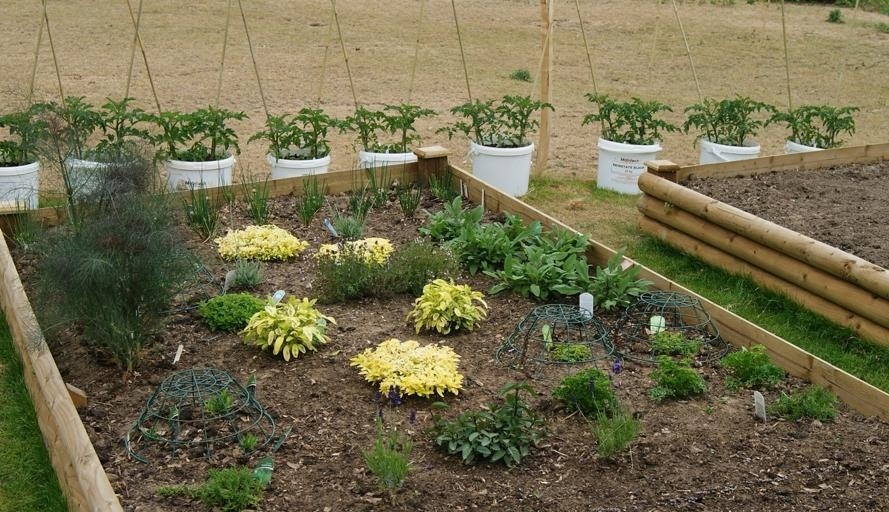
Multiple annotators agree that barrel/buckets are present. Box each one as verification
[263,145,331,179]
[360,143,419,168]
[66,156,148,201]
[594,137,664,196]
[164,145,236,189]
[0,162,40,214]
[698,135,760,166]
[783,140,826,154]
[472,133,534,199]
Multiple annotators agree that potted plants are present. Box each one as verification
[47,95,158,207]
[580,92,683,195]
[682,93,773,166]
[247,107,347,181]
[772,103,861,155]
[336,103,439,171]
[148,105,251,193]
[0,102,55,211]
[435,96,555,198]
